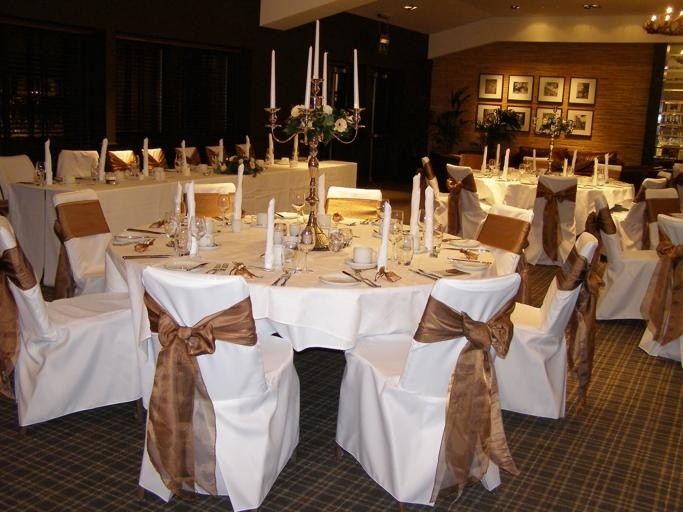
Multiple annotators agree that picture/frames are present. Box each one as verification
[506,75,533,103]
[477,72,503,100]
[568,77,597,105]
[475,103,501,130]
[535,106,562,137]
[506,105,532,134]
[565,108,594,137]
[538,76,564,104]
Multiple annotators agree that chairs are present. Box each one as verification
[0,132,681,360]
[0,217,154,433]
[476,232,607,424]
[139,264,303,512]
[335,273,521,507]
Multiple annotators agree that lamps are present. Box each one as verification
[641,0,683,39]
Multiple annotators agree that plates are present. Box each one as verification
[317,273,359,286]
[450,239,479,247]
[448,255,493,273]
[113,232,145,243]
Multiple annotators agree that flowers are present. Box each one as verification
[531,110,575,140]
[473,109,522,141]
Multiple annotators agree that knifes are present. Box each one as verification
[125,228,165,235]
[452,258,491,264]
[441,238,460,242]
[122,254,170,259]
[445,247,481,252]
[271,273,291,287]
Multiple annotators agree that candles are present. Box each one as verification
[313,20,320,79]
[269,48,277,109]
[353,48,360,108]
[304,46,313,108]
[322,53,327,105]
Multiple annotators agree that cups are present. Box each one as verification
[280,157,288,164]
[168,189,446,280]
[487,159,609,187]
[33,147,275,189]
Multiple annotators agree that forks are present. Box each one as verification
[356,274,377,288]
